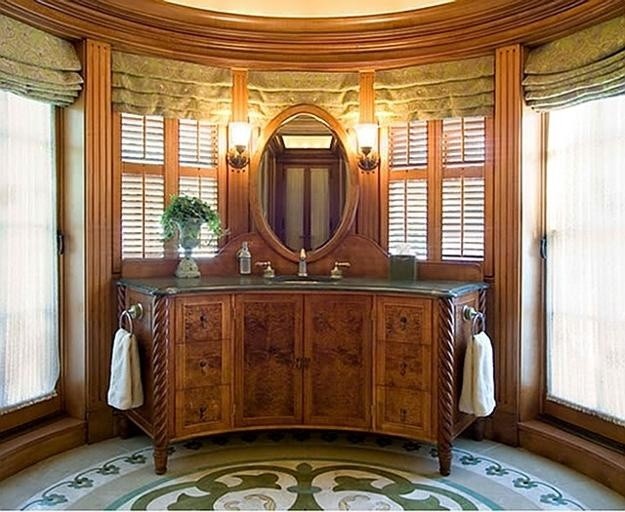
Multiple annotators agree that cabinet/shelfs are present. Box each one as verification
[233,293,375,429]
[164,294,230,430]
[376,293,439,434]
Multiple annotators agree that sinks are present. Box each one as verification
[275,274,342,286]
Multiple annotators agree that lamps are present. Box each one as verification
[355,122,380,171]
[228,122,251,168]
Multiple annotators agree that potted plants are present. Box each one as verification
[159,192,232,278]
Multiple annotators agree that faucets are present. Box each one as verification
[297,247,309,277]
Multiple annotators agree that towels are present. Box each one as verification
[459,331,498,418]
[108,329,144,411]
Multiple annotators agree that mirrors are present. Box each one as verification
[244,104,357,264]
